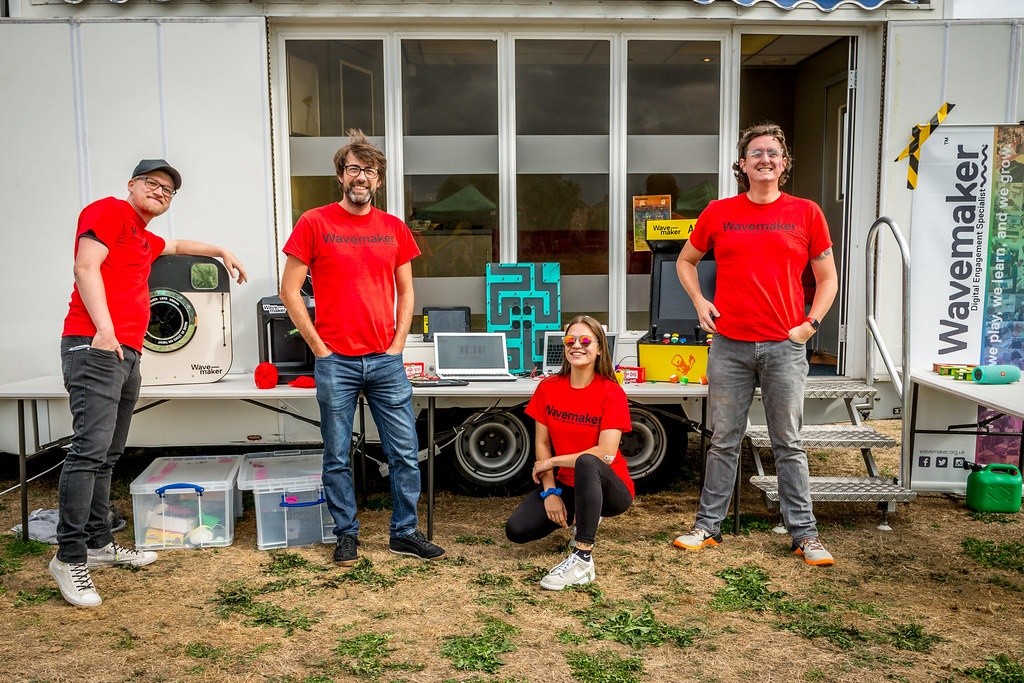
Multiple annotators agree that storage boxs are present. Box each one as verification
[238,447,344,550]
[128,452,243,548]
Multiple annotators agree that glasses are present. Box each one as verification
[745,148,785,158]
[562,334,599,346]
[341,164,380,179]
[133,177,177,198]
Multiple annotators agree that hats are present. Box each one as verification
[132,158,181,189]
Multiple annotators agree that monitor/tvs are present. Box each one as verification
[653,254,716,343]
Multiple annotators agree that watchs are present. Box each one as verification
[805,316,821,330]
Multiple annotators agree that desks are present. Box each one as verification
[0,370,742,540]
[895,360,1024,505]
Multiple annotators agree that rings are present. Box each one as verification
[533,468,534,470]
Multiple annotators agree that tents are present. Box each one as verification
[416,183,496,230]
[676,181,717,209]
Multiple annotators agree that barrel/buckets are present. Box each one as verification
[964,462,1022,513]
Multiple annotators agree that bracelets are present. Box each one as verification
[540,488,562,499]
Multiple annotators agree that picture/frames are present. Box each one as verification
[287,49,321,137]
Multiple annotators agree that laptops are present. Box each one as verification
[544,332,618,380]
[434,332,517,382]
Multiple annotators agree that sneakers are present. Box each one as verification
[389,530,446,559]
[791,536,834,565]
[573,516,603,533]
[673,525,723,550]
[333,534,360,566]
[87,539,158,570]
[49,554,103,606]
[540,549,596,590]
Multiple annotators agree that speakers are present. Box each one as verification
[257,297,315,385]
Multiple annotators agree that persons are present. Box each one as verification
[409,229,433,278]
[506,315,635,591]
[279,127,447,566]
[48,161,248,606]
[673,123,839,566]
[629,174,689,274]
